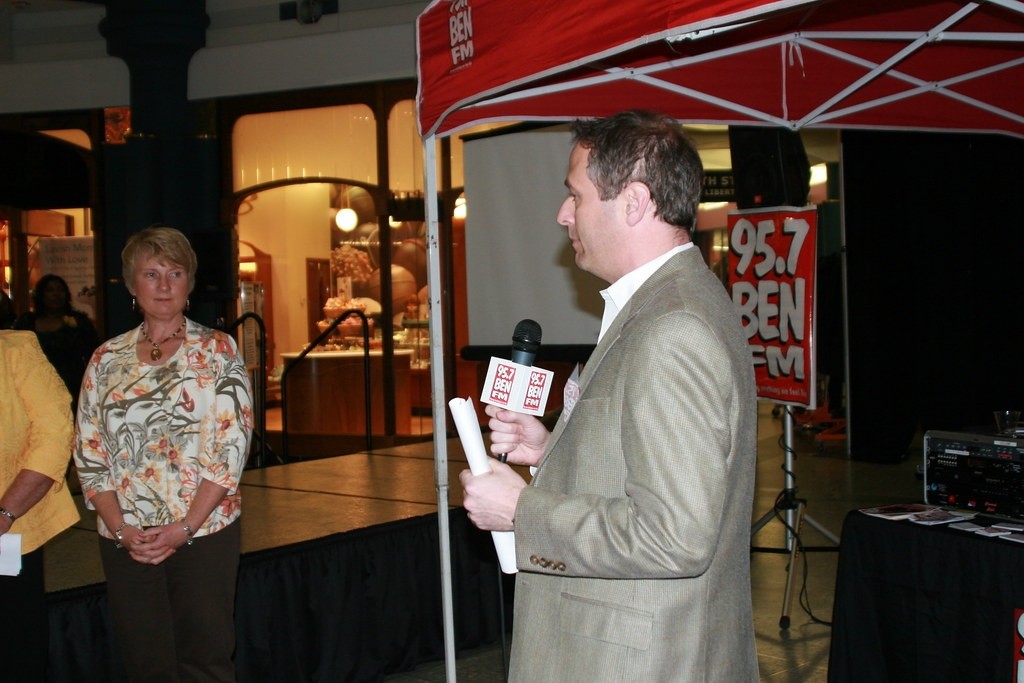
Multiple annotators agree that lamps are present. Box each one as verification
[333,185,358,235]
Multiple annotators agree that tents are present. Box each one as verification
[413,0,1024,683]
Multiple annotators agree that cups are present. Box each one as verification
[994,411,1022,435]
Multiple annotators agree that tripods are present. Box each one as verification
[748,402,841,631]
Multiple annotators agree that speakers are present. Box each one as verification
[727,124,812,210]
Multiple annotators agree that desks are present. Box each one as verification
[42,433,534,683]
[827,502,1024,683]
[279,350,414,434]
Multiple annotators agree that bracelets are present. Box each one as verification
[181,518,193,545]
[0,507,17,523]
[112,521,131,541]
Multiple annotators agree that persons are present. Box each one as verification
[459,108,762,683]
[0,273,99,682]
[73,225,255,682]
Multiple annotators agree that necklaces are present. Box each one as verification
[141,322,185,360]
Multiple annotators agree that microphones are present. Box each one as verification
[479,318,556,468]
